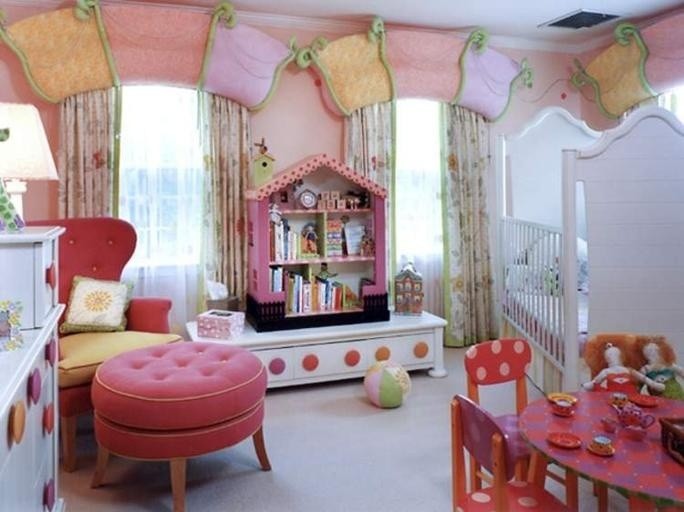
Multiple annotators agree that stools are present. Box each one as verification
[89,341,271,512]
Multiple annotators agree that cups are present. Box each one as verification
[609,393,627,407]
[592,436,612,451]
[557,399,572,413]
[548,393,577,404]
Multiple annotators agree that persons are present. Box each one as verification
[630,334,684,403]
[579,333,665,409]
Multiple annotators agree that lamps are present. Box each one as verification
[0,102,60,224]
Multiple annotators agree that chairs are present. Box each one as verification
[464,338,578,512]
[584,334,637,512]
[450,393,570,512]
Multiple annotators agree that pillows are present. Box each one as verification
[556,255,589,296]
[59,274,135,334]
[505,264,558,296]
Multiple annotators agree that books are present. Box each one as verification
[269,219,367,315]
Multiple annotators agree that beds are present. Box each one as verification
[494,104,684,399]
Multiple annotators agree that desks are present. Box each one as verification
[184,305,450,389]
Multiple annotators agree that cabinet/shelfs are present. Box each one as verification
[246,153,390,333]
[0,303,66,512]
[0,226,67,331]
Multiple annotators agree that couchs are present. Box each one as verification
[25,217,185,473]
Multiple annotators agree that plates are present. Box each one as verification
[546,431,583,449]
[629,396,661,405]
[552,410,574,416]
[588,444,616,456]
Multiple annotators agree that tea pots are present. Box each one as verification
[613,402,655,428]
